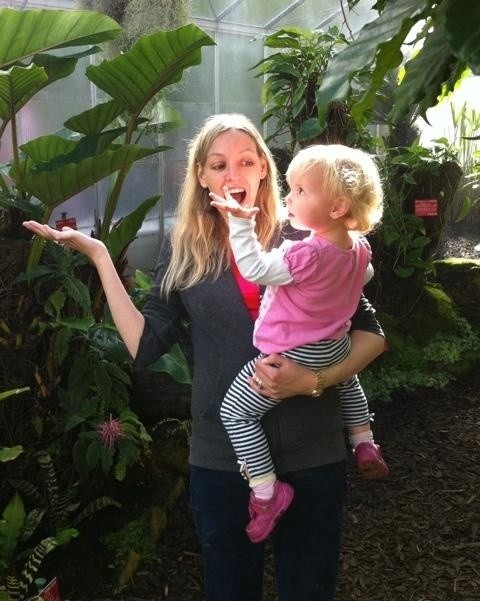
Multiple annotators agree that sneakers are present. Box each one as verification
[246,481,295,544]
[352,443,389,480]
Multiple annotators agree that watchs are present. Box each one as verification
[311,369,326,399]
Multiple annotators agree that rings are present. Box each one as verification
[258,379,264,389]
[256,376,260,384]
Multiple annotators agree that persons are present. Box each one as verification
[23,110,389,600]
[207,142,391,545]
